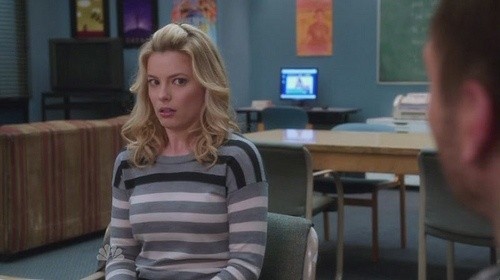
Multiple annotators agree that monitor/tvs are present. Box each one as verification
[48,38,123,91]
[279,68,319,106]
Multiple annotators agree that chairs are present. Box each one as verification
[80,106,496,279]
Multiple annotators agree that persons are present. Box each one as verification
[105,22,269,280]
[424,0,500,280]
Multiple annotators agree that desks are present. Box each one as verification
[242,126,463,280]
[41,88,131,121]
[237,105,361,134]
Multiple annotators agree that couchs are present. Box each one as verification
[0,114,131,260]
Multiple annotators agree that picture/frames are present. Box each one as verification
[116,0,159,48]
[377,0,446,86]
[70,0,109,38]
